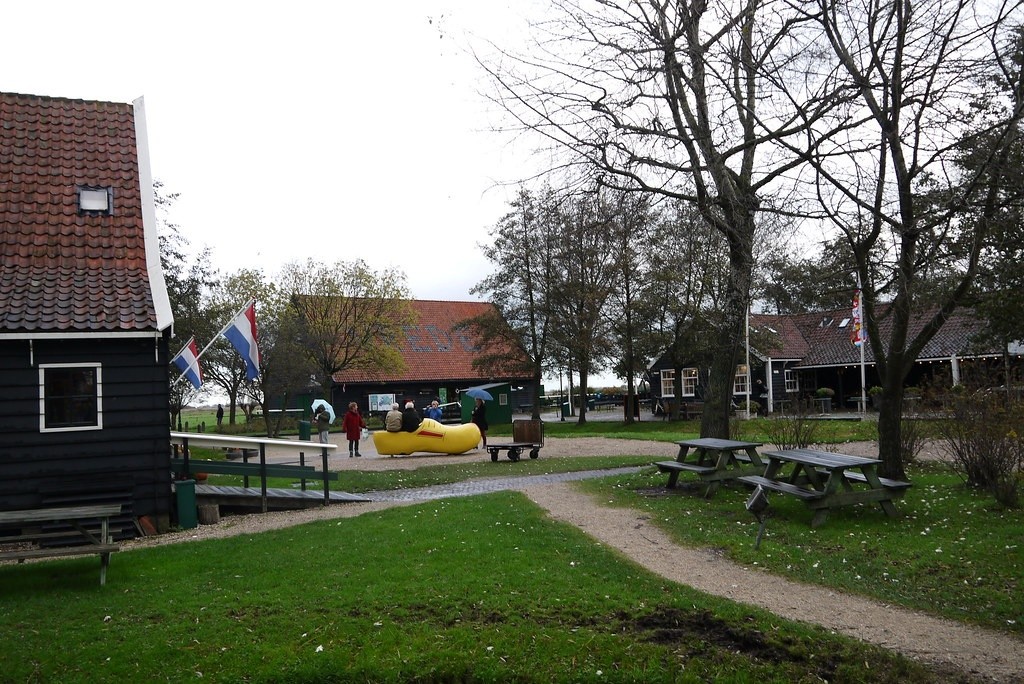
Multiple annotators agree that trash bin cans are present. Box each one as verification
[563,402,569,417]
[298,419,311,440]
[174,479,197,529]
[589,399,595,411]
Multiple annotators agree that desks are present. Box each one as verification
[666,438,764,498]
[761,449,898,528]
[0,504,121,585]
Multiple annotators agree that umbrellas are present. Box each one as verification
[311,399,336,424]
[465,388,493,400]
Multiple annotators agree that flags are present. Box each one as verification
[173,339,203,388]
[223,302,264,381]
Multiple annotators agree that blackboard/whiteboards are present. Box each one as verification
[624,395,640,417]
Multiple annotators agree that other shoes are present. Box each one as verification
[355,451,362,458]
[349,451,354,457]
[482,446,488,449]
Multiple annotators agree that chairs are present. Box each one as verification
[661,401,684,421]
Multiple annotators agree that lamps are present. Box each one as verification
[744,485,769,524]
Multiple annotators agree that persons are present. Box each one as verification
[424,401,442,423]
[316,404,330,456]
[342,401,365,456]
[471,398,488,448]
[434,396,443,404]
[403,401,422,432]
[384,403,402,432]
[216,404,224,425]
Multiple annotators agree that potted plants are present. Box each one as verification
[868,387,883,410]
[735,401,761,420]
[816,388,835,412]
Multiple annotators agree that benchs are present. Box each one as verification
[687,404,704,420]
[0,527,122,560]
[652,437,913,530]
